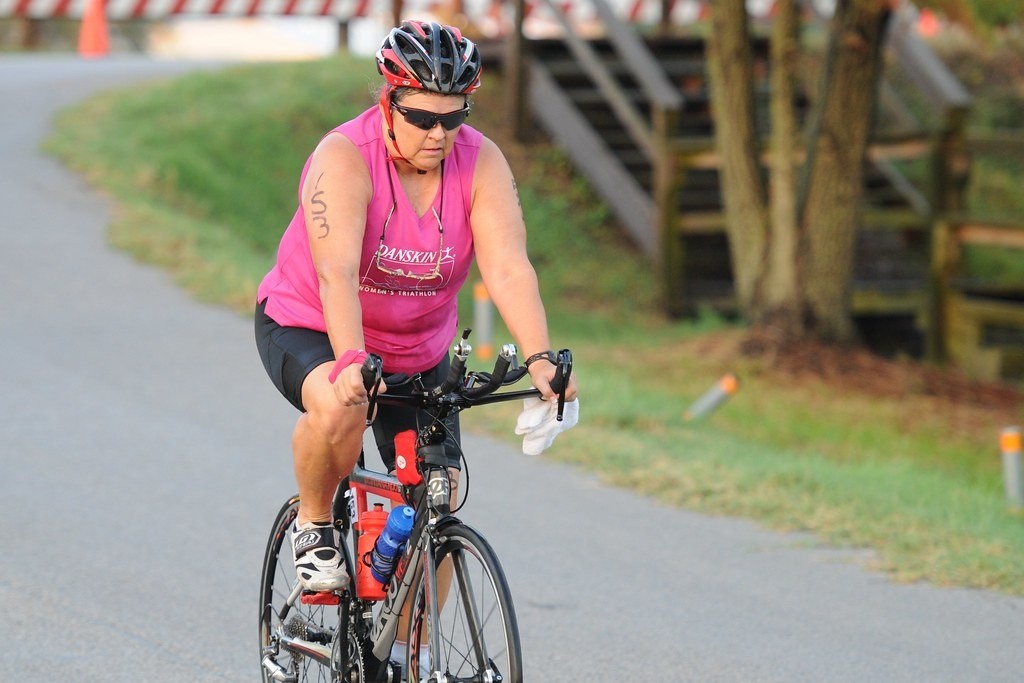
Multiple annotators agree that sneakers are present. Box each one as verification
[289,511,351,592]
[387,667,429,683]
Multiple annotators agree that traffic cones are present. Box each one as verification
[77,0,110,61]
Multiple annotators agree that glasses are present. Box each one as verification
[390,100,472,131]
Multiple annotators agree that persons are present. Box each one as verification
[252,19,579,682]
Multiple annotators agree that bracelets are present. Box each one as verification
[525,350,557,369]
[328,349,369,384]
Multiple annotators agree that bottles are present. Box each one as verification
[372,506,416,584]
[359,503,389,600]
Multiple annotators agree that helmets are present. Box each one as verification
[376,20,482,94]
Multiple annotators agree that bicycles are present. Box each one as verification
[257,324,573,683]
